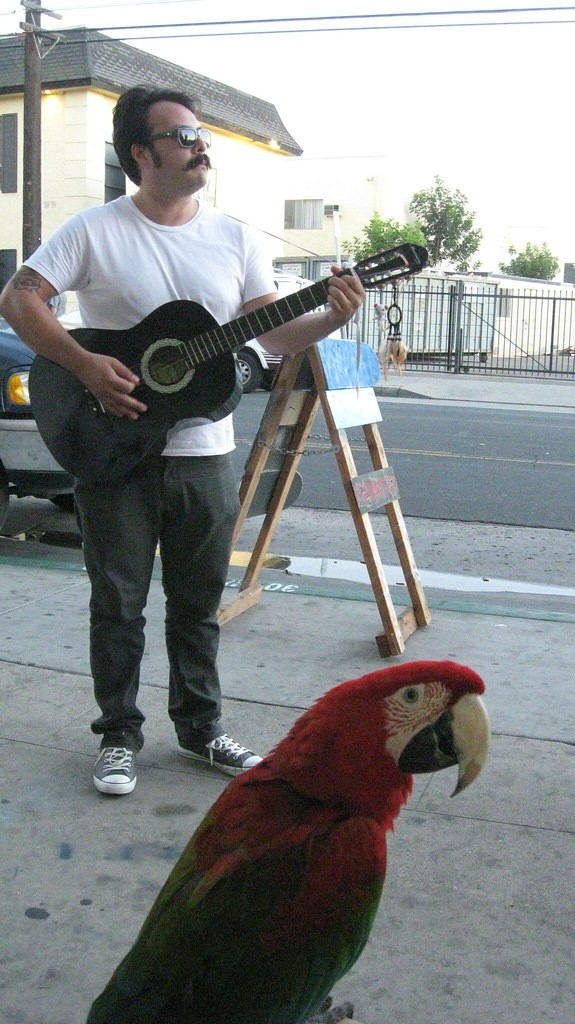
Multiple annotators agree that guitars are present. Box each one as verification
[26,243,430,485]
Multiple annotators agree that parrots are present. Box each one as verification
[86,661,490,1024]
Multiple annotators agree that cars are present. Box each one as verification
[0,329,82,532]
[57,267,324,393]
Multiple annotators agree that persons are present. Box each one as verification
[0,85,365,794]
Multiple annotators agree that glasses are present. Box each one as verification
[142,127,213,148]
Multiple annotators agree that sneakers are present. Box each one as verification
[177,735,264,776]
[92,745,138,794]
